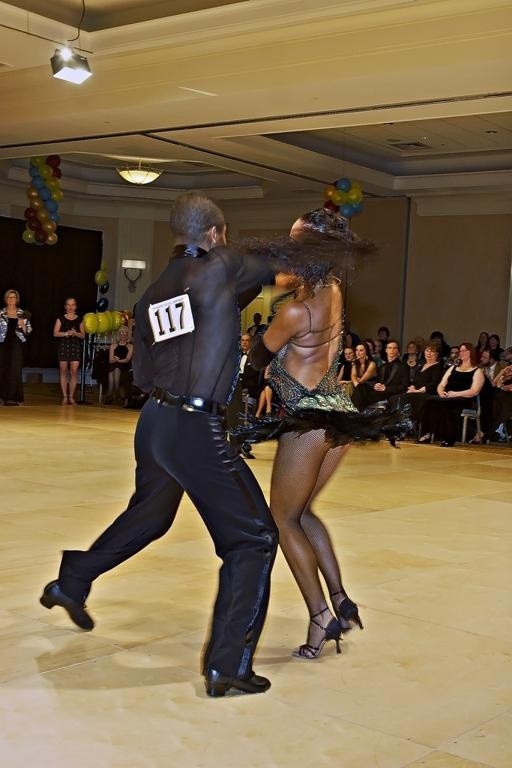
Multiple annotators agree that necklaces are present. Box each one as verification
[316,275,342,286]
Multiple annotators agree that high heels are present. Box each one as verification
[397,432,410,441]
[330,590,363,634]
[294,608,341,660]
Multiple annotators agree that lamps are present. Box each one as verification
[121,259,147,292]
[116,159,165,185]
[0,0,93,85]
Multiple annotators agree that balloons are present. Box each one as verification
[22,155,64,247]
[325,177,364,219]
[82,261,130,334]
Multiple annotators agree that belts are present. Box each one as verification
[149,388,226,419]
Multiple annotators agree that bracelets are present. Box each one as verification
[65,332,68,337]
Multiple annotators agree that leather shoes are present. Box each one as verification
[441,441,453,447]
[40,580,93,630]
[415,436,431,444]
[206,670,272,697]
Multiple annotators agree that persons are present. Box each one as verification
[50,297,86,406]
[39,193,302,698]
[0,289,33,405]
[226,209,364,659]
[104,313,512,445]
